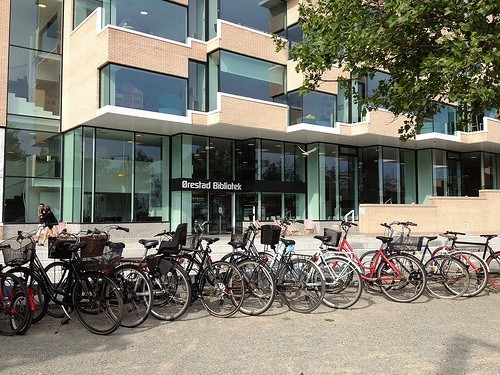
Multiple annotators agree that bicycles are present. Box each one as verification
[0,220,500,337]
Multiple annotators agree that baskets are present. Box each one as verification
[261,225,281,244]
[324,228,342,247]
[79,235,108,258]
[231,227,252,246]
[180,234,196,254]
[0,235,35,266]
[389,236,423,251]
[48,237,72,259]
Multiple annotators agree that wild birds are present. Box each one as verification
[298,145,319,157]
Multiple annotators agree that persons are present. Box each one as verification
[36,203,60,246]
